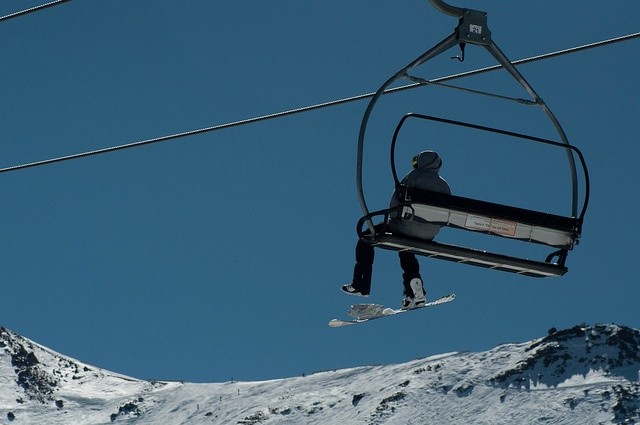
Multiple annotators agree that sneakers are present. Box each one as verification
[403,278,426,308]
[341,284,370,298]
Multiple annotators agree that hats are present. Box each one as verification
[412,149,442,173]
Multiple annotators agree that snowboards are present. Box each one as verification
[329,295,455,327]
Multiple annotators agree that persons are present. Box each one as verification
[340,149,451,310]
